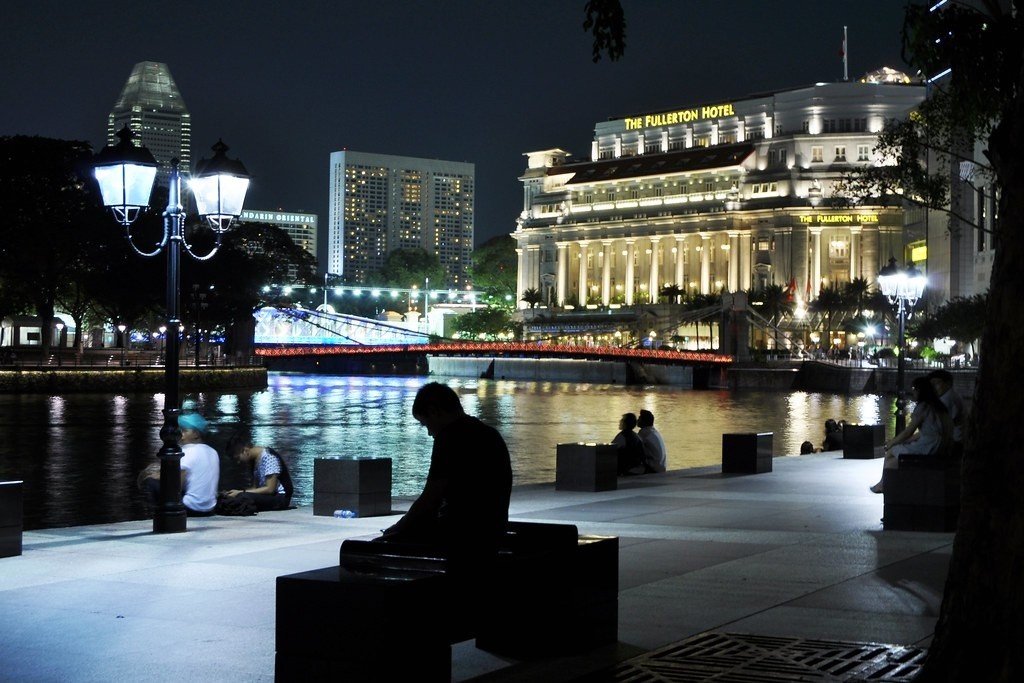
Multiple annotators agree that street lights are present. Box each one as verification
[878,254,927,441]
[90,122,253,532]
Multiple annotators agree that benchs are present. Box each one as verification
[881,451,961,532]
[274,520,619,682]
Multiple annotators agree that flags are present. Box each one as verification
[787,271,811,300]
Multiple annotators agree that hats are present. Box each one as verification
[179,412,208,433]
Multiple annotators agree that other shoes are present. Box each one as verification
[870,481,884,492]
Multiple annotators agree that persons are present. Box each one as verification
[635,409,667,472]
[370,380,512,560]
[870,377,953,494]
[612,412,646,477]
[139,413,221,517]
[798,418,849,456]
[904,368,969,455]
[219,433,292,513]
[806,340,861,365]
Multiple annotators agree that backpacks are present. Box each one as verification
[216,492,260,517]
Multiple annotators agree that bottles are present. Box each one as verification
[334,510,357,518]
